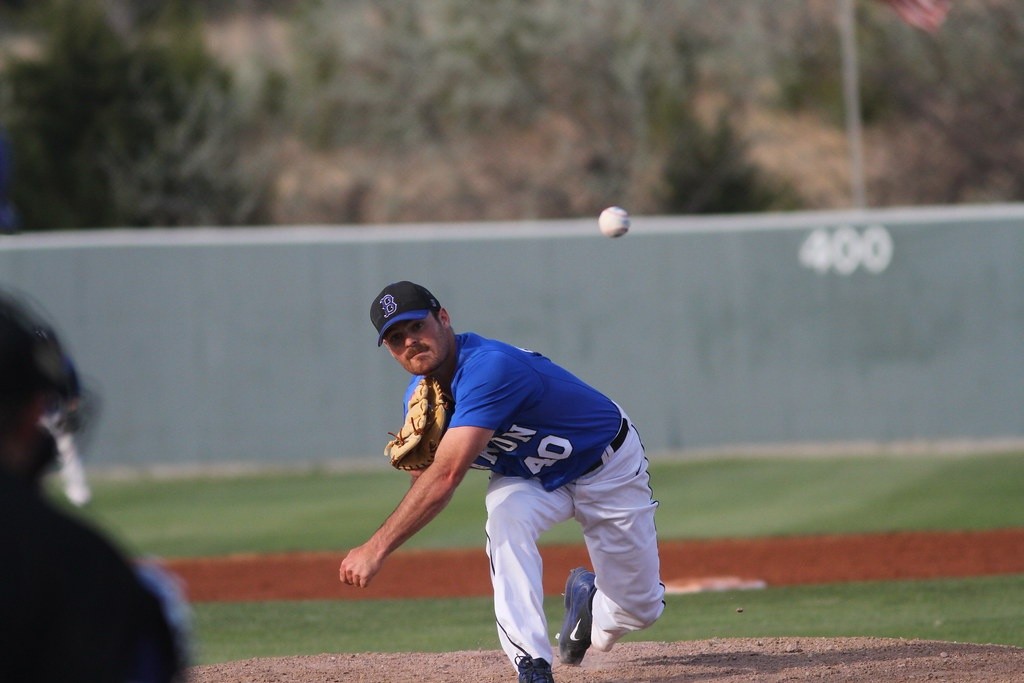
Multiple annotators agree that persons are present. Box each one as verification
[340,282,666,683]
[0,287,195,683]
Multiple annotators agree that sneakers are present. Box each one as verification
[514,654,554,683]
[555,566,598,666]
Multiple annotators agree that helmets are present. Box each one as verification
[0,288,85,472]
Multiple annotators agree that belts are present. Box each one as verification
[581,418,629,477]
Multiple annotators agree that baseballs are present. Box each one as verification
[597,205,633,238]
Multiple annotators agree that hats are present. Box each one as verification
[370,280,441,347]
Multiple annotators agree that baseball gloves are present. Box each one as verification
[384,375,449,471]
